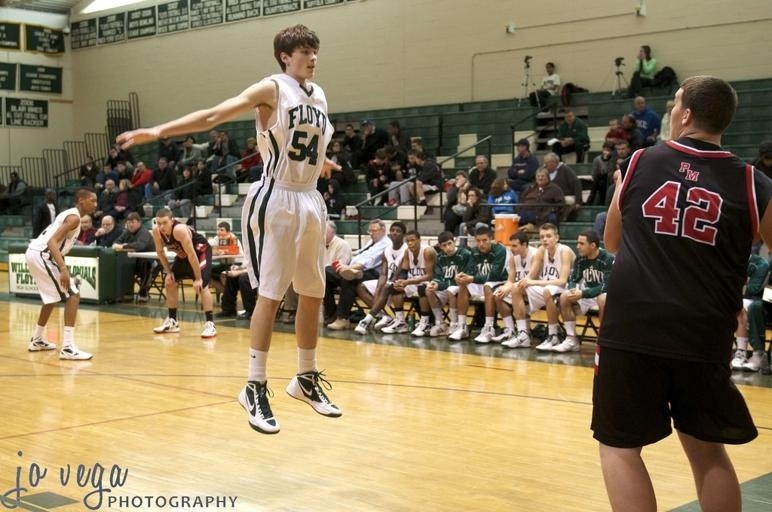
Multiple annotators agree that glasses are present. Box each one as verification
[367,229,382,234]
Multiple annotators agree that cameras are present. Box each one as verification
[614,57,626,67]
[523,55,533,66]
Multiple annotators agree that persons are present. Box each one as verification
[0,43,772,377]
[113,23,343,434]
[588,74,772,512]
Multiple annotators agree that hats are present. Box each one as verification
[513,138,529,146]
[361,119,372,126]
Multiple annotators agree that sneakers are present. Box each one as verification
[283,316,295,324]
[381,320,409,334]
[536,335,561,351]
[446,325,457,335]
[760,364,772,375]
[152,317,180,333]
[743,353,768,370]
[237,380,281,435]
[490,331,516,343]
[200,320,217,338]
[285,371,343,418]
[449,324,469,340]
[474,326,496,343]
[27,336,56,352]
[237,311,253,320]
[501,331,531,348]
[731,356,748,369]
[58,346,93,360]
[552,336,580,353]
[428,324,449,337]
[374,316,395,330]
[411,323,431,337]
[355,320,368,335]
[137,295,149,302]
[215,309,237,318]
[326,317,350,330]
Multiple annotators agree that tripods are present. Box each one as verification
[610,67,633,99]
[517,67,543,108]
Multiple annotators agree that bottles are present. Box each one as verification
[408,311,415,333]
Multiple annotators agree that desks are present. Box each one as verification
[6,240,135,305]
